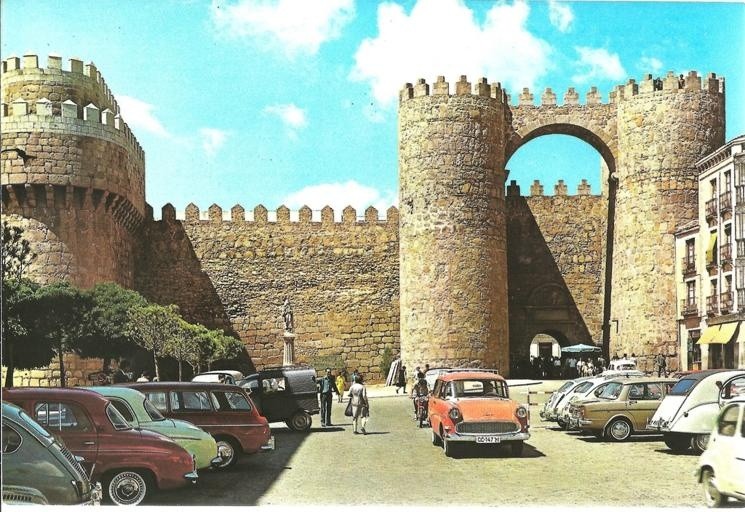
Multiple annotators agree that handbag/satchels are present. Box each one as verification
[344,398,352,416]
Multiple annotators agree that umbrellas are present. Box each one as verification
[562,344,602,366]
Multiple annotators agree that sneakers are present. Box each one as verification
[360,427,366,435]
[322,421,332,427]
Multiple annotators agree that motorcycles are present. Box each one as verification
[410,389,433,427]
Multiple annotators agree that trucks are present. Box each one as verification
[106,369,322,471]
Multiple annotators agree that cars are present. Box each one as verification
[70,387,223,470]
[0,398,103,505]
[646,368,745,457]
[428,371,531,457]
[540,359,693,443]
[1,386,198,506]
[422,368,486,395]
[694,394,745,508]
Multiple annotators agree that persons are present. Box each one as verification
[218,373,226,384]
[396,364,430,421]
[348,375,368,434]
[114,361,130,382]
[657,353,668,378]
[281,299,293,333]
[352,369,359,383]
[335,372,346,403]
[530,352,637,379]
[136,371,149,382]
[317,368,340,427]
